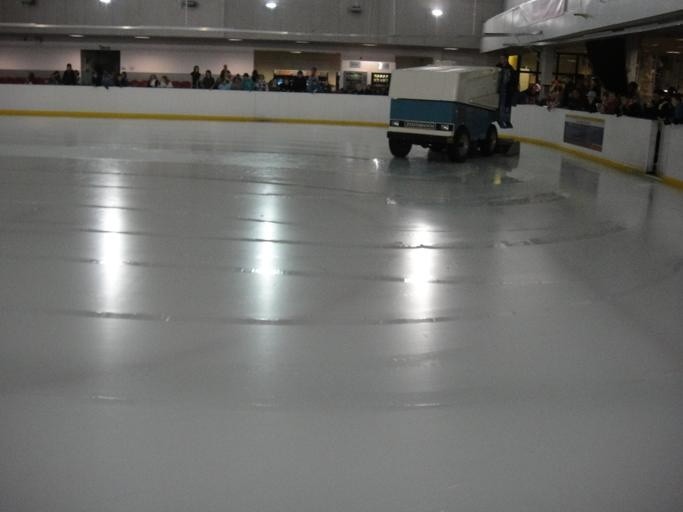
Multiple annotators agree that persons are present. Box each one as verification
[20,57,390,96]
[516,73,682,126]
[494,51,514,73]
